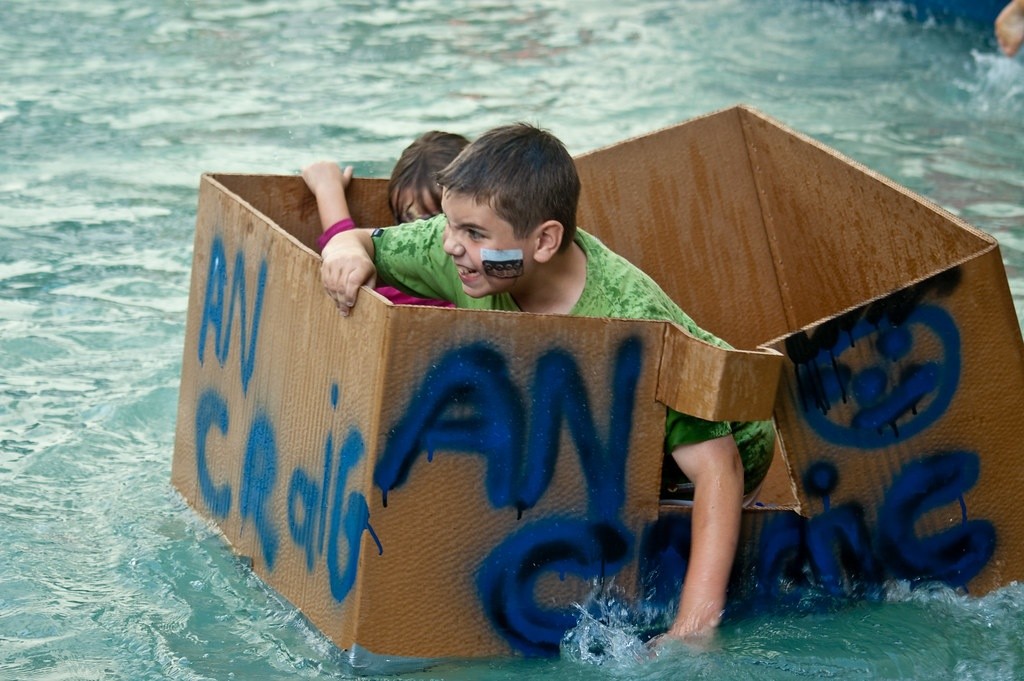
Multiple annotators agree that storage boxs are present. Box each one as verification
[167,106,1024,680]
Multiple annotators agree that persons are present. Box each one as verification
[300,131,473,307]
[322,123,777,653]
[993,0,1024,54]
